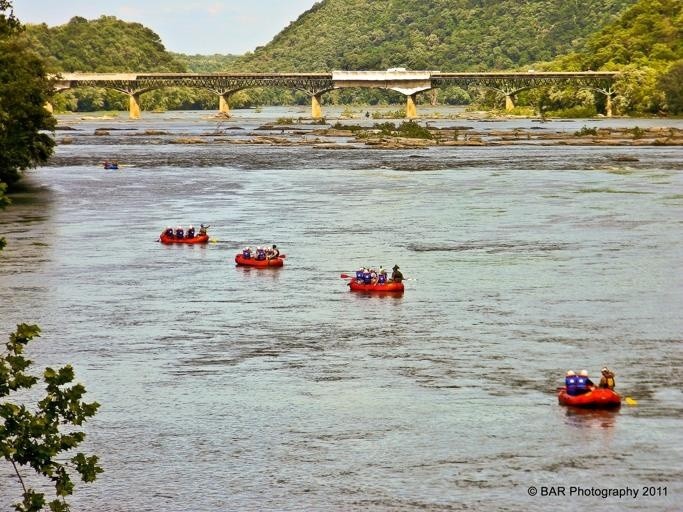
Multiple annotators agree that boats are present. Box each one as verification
[557,386,620,410]
[160,232,207,244]
[349,278,403,292]
[104,165,118,170]
[235,254,282,268]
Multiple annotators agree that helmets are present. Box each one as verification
[566,370,589,377]
[360,268,384,277]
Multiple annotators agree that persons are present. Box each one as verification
[355,264,405,285]
[241,244,280,263]
[164,223,211,240]
[104,160,118,168]
[564,366,616,396]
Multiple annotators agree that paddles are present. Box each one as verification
[340,274,355,279]
[613,389,636,405]
[277,255,285,259]
